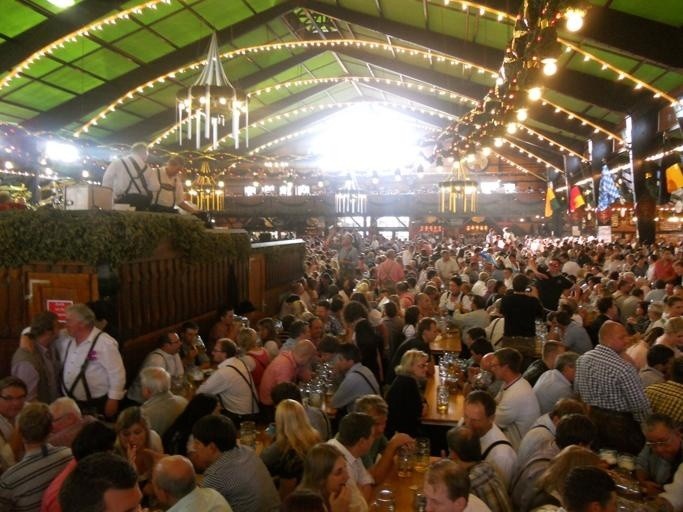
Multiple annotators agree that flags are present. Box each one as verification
[569,186,586,213]
[598,165,621,212]
[544,188,559,217]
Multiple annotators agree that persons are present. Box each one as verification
[127,331,184,407]
[353,395,415,470]
[457,391,517,490]
[391,318,437,376]
[345,301,386,385]
[565,467,617,512]
[46,397,99,447]
[162,393,222,455]
[270,382,331,442]
[327,413,376,502]
[260,399,322,495]
[533,353,581,414]
[113,407,164,482]
[195,338,259,428]
[653,461,683,512]
[280,315,296,343]
[308,316,322,347]
[102,142,159,212]
[526,444,602,511]
[638,344,675,386]
[280,320,310,351]
[574,320,652,457]
[19,304,126,417]
[518,397,585,469]
[152,455,233,512]
[512,414,595,510]
[319,336,339,362]
[332,343,380,412]
[257,318,280,356]
[644,356,683,427]
[178,322,206,369]
[314,301,342,335]
[0,376,28,443]
[490,347,540,447]
[59,452,144,512]
[40,421,117,512]
[151,156,195,213]
[240,222,436,317]
[140,367,189,435]
[424,458,492,512]
[10,311,62,403]
[0,403,73,512]
[237,328,270,389]
[190,413,282,512]
[282,443,352,512]
[436,222,683,355]
[446,425,513,512]
[480,352,503,396]
[210,305,240,344]
[85,298,123,346]
[383,349,429,441]
[261,340,317,405]
[635,413,683,492]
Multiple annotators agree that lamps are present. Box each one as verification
[217,178,225,189]
[562,8,585,32]
[82,168,90,178]
[539,58,559,78]
[492,137,505,148]
[505,121,518,134]
[334,168,368,215]
[176,31,251,153]
[184,177,192,187]
[653,213,661,223]
[286,177,294,188]
[185,160,225,211]
[3,160,15,170]
[252,176,260,186]
[514,108,530,122]
[438,159,479,212]
[631,213,638,222]
[527,87,544,101]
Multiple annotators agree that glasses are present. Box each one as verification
[0,394,26,401]
[646,434,673,447]
[417,362,429,368]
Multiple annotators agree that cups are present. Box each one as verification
[414,439,429,472]
[396,445,413,477]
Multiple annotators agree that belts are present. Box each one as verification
[593,406,632,417]
